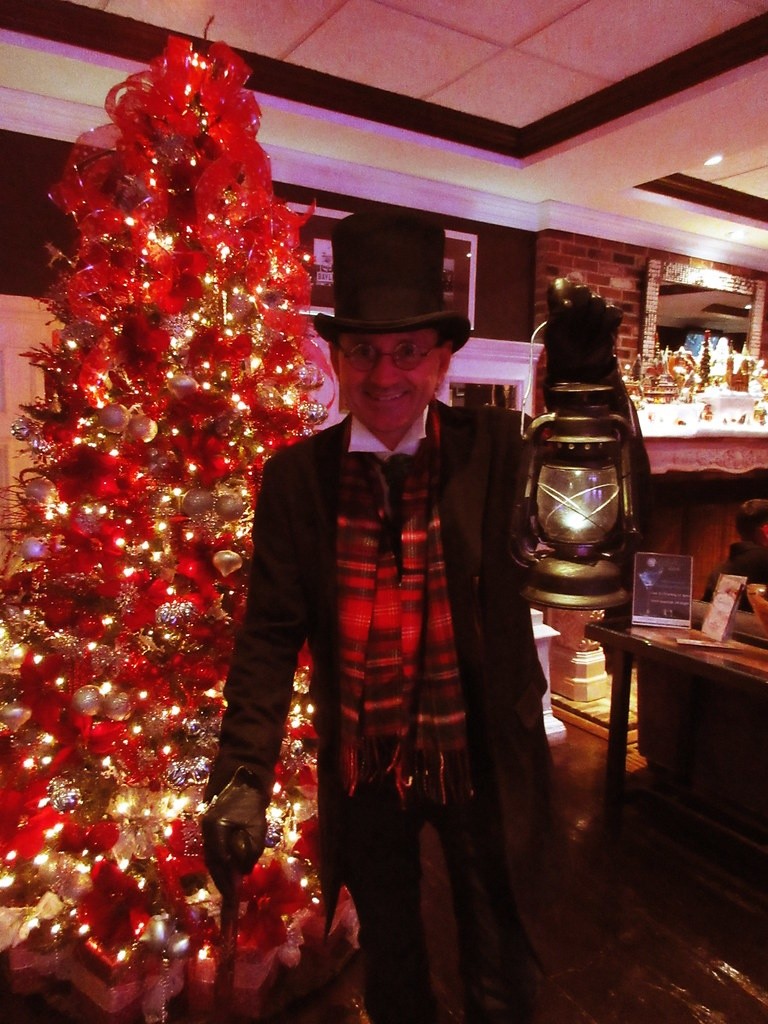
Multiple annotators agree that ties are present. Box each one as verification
[380,453,409,585]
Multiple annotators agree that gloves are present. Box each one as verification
[545,277,624,385]
[200,776,268,874]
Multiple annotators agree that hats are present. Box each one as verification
[313,212,471,353]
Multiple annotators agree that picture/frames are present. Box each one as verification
[285,202,479,331]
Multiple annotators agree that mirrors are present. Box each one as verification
[584,616,768,889]
[642,257,766,359]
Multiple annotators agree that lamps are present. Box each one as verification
[515,320,639,611]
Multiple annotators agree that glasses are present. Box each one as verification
[331,340,445,372]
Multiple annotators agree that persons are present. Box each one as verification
[202,211,625,1024]
[702,499,768,613]
